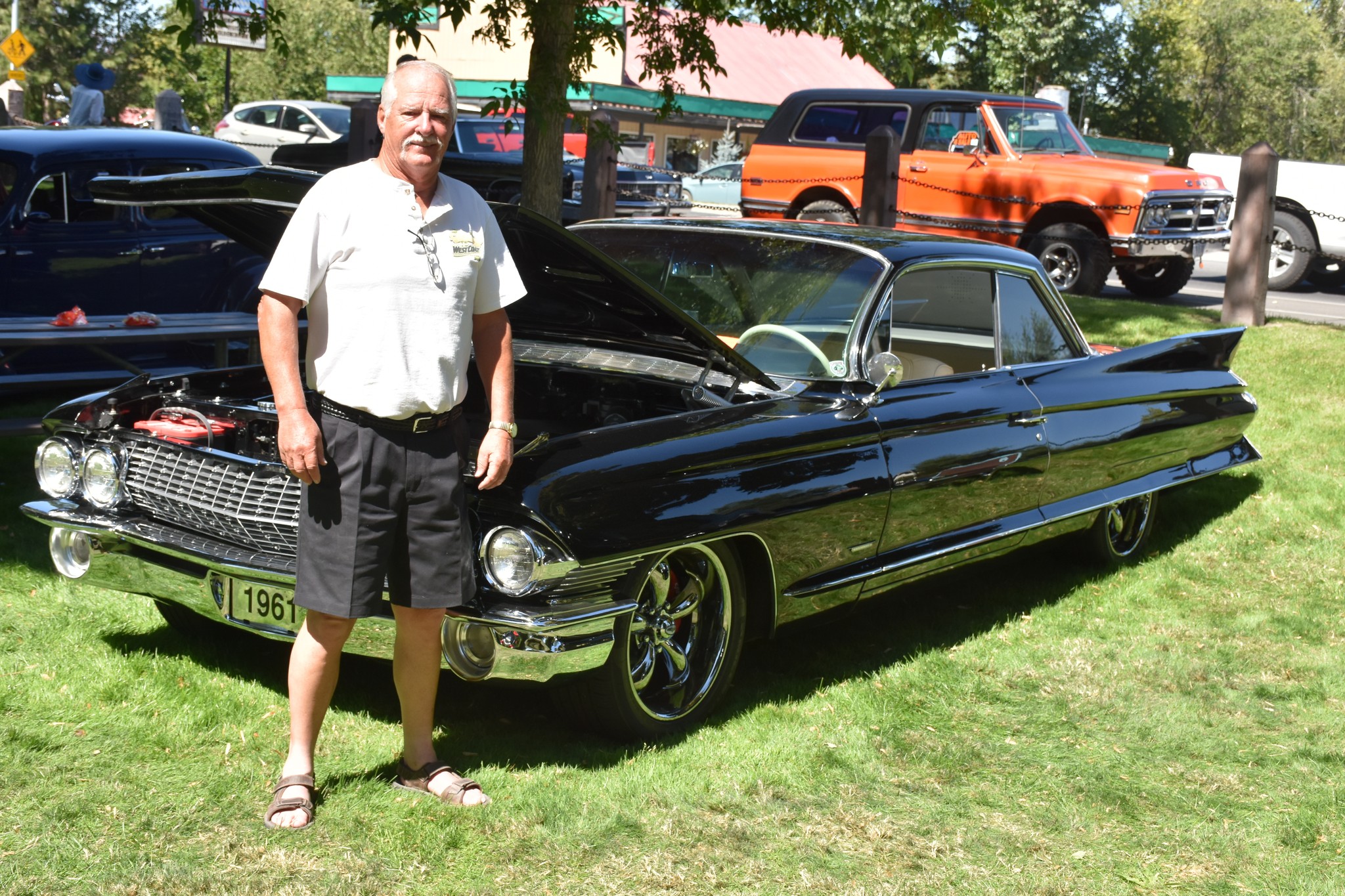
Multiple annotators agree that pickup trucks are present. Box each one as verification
[1186,152,1345,290]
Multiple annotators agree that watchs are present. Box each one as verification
[489,420,518,438]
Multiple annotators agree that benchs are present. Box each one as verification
[711,328,956,383]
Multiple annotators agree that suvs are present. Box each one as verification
[743,86,1234,300]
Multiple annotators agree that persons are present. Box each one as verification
[66,62,116,129]
[259,60,527,830]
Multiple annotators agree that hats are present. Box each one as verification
[74,62,116,90]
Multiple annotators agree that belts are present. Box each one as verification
[309,393,463,434]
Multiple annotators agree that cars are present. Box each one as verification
[1,166,347,465]
[676,161,744,207]
[271,103,695,226]
[0,126,280,314]
[19,202,1265,737]
[212,100,362,170]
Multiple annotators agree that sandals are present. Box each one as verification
[392,751,492,806]
[263,772,315,830]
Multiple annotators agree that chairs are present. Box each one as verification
[248,111,266,127]
[282,109,302,132]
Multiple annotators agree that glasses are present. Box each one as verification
[406,220,443,284]
